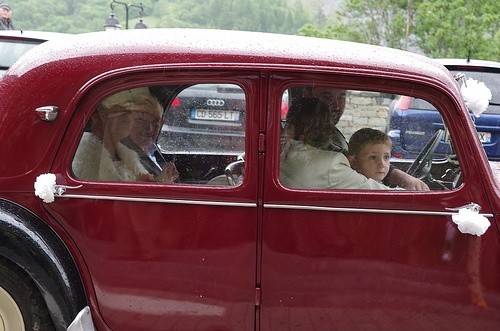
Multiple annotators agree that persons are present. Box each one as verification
[72,88,179,186]
[0,4,14,30]
[279,85,432,192]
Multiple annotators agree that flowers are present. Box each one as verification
[135,172,155,183]
[34,173,82,203]
[460,77,492,118]
[452,203,491,237]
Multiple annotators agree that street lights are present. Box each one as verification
[103,0,147,31]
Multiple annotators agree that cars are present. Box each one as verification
[158,84,289,155]
[384,51,500,189]
[0,28,500,331]
[0,30,67,78]
